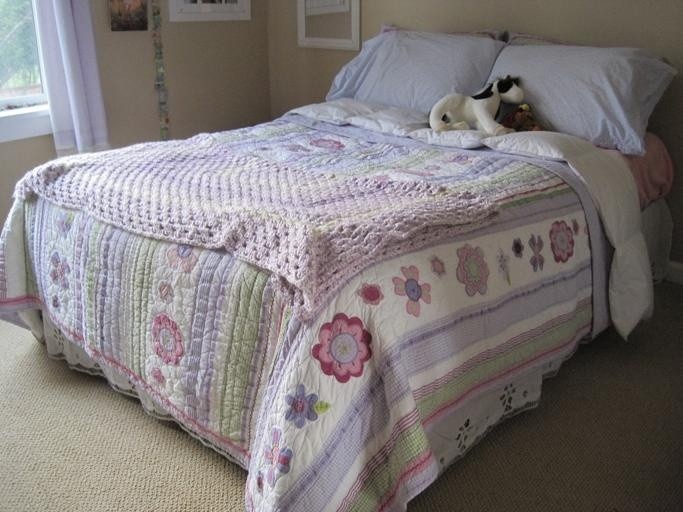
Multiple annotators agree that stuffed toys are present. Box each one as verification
[500,103,540,132]
[427,74,524,136]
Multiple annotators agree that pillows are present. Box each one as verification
[326,26,676,155]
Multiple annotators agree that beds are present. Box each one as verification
[20,101,672,487]
